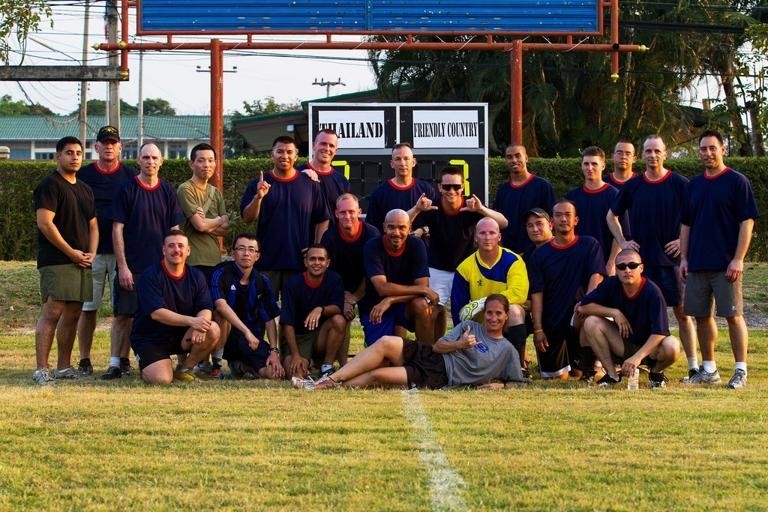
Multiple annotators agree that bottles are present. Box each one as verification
[628,369,639,391]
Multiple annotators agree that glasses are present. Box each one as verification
[234,247,259,254]
[616,261,643,271]
[441,183,463,192]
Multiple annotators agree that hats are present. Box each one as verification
[98,126,120,142]
[527,208,551,220]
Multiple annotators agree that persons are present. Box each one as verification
[243,128,690,381]
[292,294,523,390]
[575,250,681,390]
[33,134,100,385]
[100,141,185,378]
[208,233,284,383]
[130,229,220,385]
[177,141,229,373]
[679,129,757,388]
[76,126,139,380]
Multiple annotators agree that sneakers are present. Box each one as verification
[291,376,344,392]
[102,366,122,381]
[320,364,333,375]
[520,367,533,380]
[727,367,749,389]
[592,373,621,389]
[31,369,56,385]
[174,370,203,384]
[54,368,82,380]
[79,358,95,377]
[122,357,134,375]
[647,369,666,391]
[677,367,721,385]
